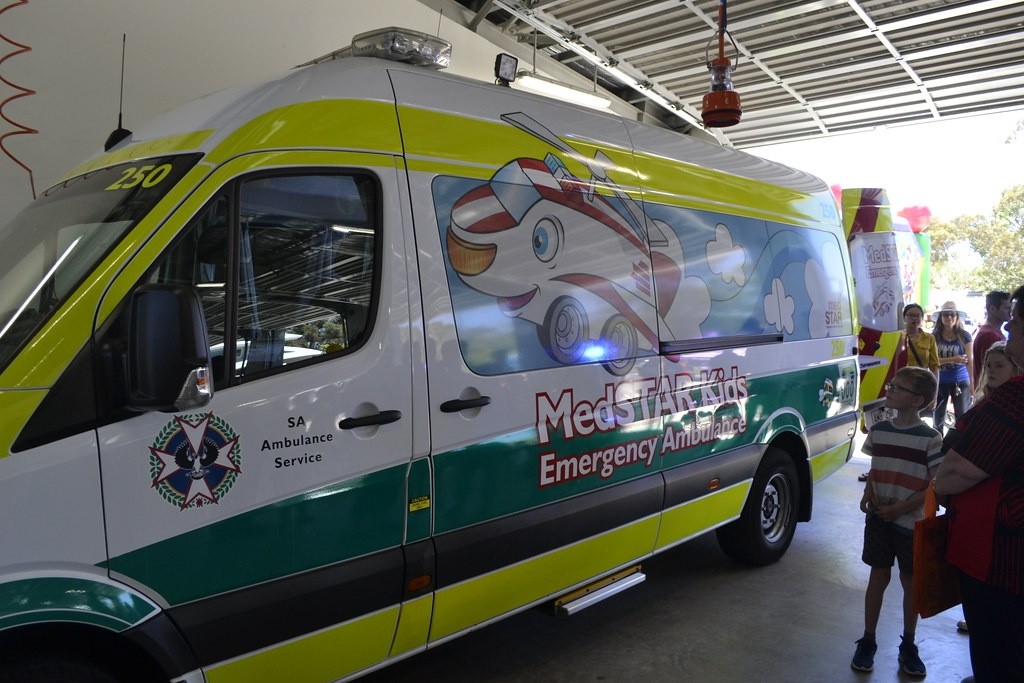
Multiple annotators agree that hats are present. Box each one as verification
[931,300,968,323]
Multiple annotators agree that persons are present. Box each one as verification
[972,291,1012,389]
[930,302,975,439]
[858,303,940,483]
[851,365,943,676]
[974,341,1016,397]
[931,286,1024,683]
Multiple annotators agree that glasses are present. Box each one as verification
[883,381,920,398]
[906,312,923,318]
[941,312,957,317]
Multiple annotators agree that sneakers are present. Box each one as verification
[851,638,878,671]
[957,619,968,631]
[961,675,975,683]
[898,635,927,676]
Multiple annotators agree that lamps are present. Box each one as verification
[516,27,613,108]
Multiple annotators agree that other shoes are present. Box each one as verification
[857,472,870,481]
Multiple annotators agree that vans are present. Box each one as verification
[0,24,912,683]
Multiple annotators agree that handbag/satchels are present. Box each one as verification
[912,478,962,618]
